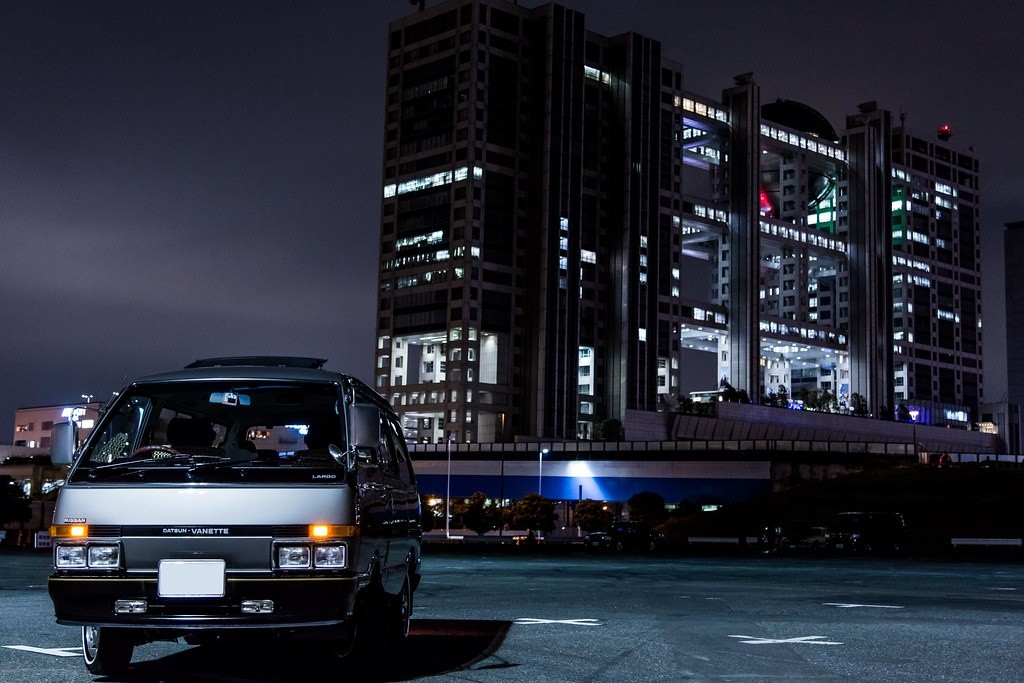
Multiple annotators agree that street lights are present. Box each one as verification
[538,448,549,542]
[446,432,456,537]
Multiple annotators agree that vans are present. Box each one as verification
[864,508,954,562]
[47,355,423,677]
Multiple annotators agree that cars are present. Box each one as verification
[650,512,775,563]
[582,528,625,557]
[814,525,872,557]
[775,519,819,558]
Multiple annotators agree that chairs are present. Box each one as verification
[257,449,281,467]
[307,422,339,450]
[217,441,258,460]
[165,417,216,449]
